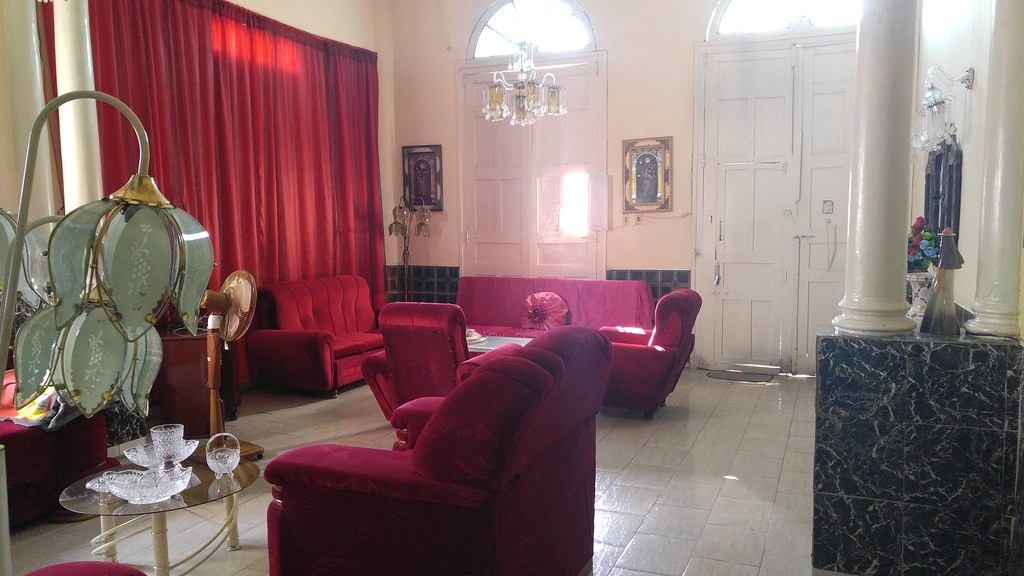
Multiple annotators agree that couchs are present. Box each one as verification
[455,275,655,340]
[233,275,390,399]
[265,326,617,575]
[0,331,122,537]
[22,559,150,576]
[360,301,471,423]
[262,323,614,576]
[598,287,703,421]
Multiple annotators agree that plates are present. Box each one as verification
[85,475,201,493]
[466,336,487,344]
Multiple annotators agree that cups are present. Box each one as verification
[149,423,184,471]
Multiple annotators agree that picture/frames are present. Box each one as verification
[399,144,447,213]
[618,135,677,216]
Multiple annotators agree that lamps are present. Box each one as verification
[387,193,433,302]
[909,60,976,154]
[0,89,216,575]
[481,39,570,128]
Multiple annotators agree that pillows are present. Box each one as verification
[519,290,572,331]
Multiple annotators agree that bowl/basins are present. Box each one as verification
[467,329,475,337]
[123,440,199,467]
[106,466,192,504]
[205,433,240,480]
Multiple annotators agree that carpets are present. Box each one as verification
[706,370,777,382]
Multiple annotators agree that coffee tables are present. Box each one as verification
[57,456,259,576]
[461,336,534,353]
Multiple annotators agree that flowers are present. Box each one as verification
[907,216,942,273]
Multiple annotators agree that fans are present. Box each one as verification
[196,269,265,462]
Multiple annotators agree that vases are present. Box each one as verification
[906,273,936,317]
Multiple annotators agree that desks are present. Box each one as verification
[814,299,1024,575]
[139,317,219,440]
